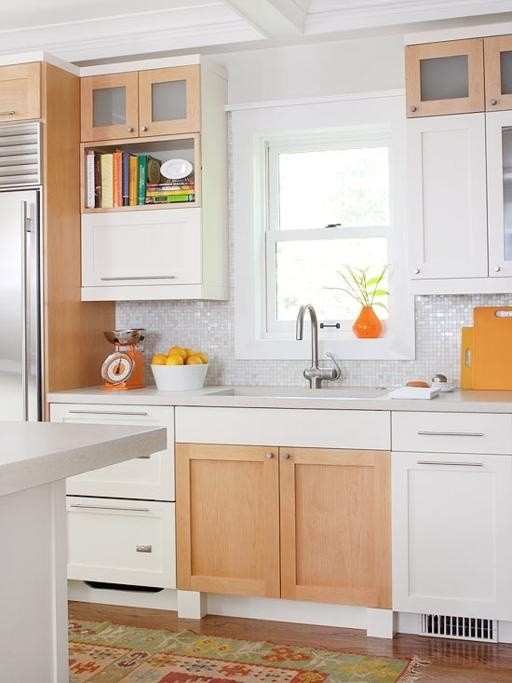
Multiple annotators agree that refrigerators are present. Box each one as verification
[0,188,42,425]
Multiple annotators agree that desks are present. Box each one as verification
[1,420,171,683]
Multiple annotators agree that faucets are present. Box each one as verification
[295,303,341,388]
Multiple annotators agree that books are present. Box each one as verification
[86,150,195,208]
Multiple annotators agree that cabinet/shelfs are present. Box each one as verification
[77,53,226,301]
[174,404,393,611]
[50,401,178,592]
[0,51,116,423]
[390,409,512,623]
[404,19,512,294]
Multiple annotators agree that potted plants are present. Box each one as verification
[322,259,394,337]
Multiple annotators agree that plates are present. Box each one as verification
[160,158,192,180]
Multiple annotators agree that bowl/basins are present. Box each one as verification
[150,362,210,393]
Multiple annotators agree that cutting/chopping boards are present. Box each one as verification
[460,305,512,391]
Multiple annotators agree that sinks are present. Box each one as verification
[204,387,391,399]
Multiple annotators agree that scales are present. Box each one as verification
[101,329,146,391]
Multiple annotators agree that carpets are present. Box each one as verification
[68,616,433,682]
